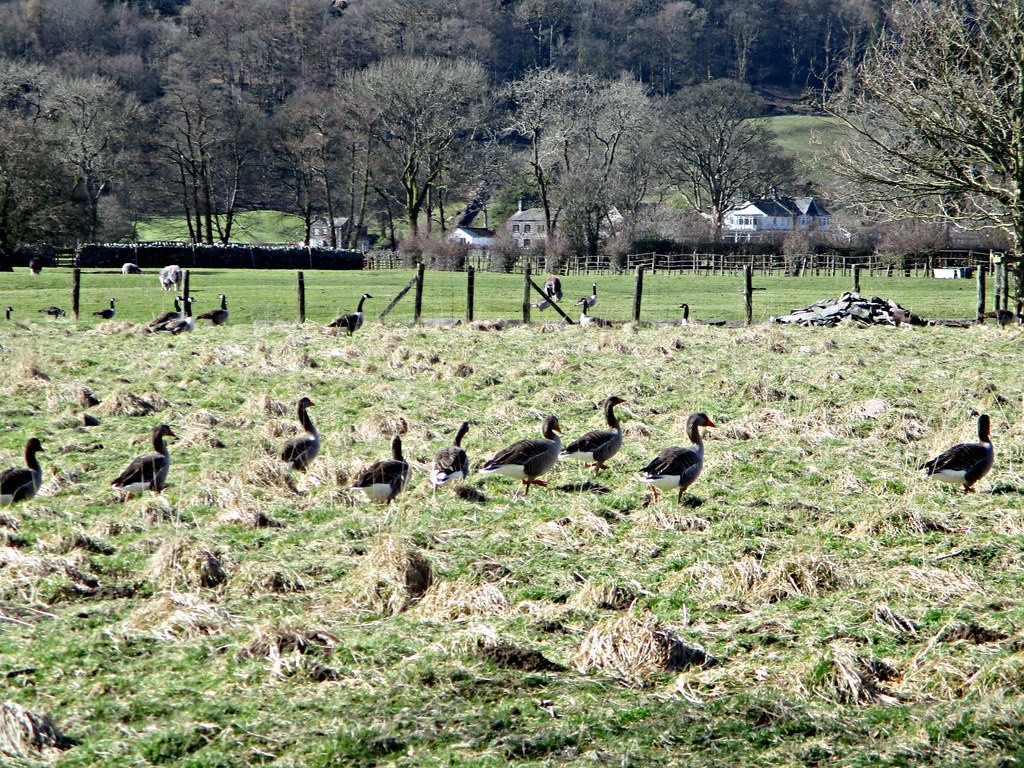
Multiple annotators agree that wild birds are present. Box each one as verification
[678,304,694,325]
[281,396,627,506]
[638,411,717,504]
[146,294,230,337]
[577,283,606,328]
[326,293,373,336]
[531,284,555,311]
[6,306,14,321]
[38,306,66,320]
[919,414,994,503]
[0,437,45,505]
[110,424,176,503]
[93,297,117,319]
